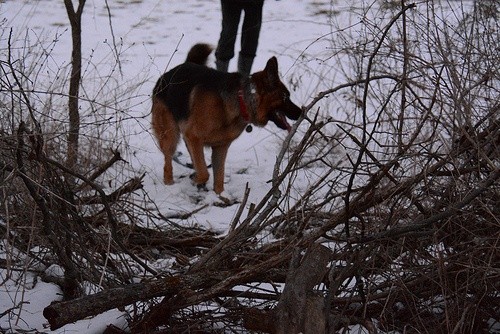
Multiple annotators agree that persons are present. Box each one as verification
[214,0,265,81]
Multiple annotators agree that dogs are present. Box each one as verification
[151,43,301,194]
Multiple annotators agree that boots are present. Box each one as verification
[237,52,256,75]
[216,58,229,72]
